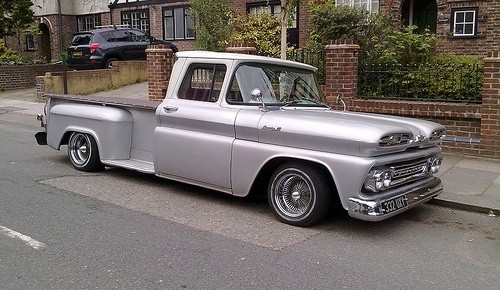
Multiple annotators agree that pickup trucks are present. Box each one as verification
[34,48,446,231]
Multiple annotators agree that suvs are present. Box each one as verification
[66,25,179,70]
